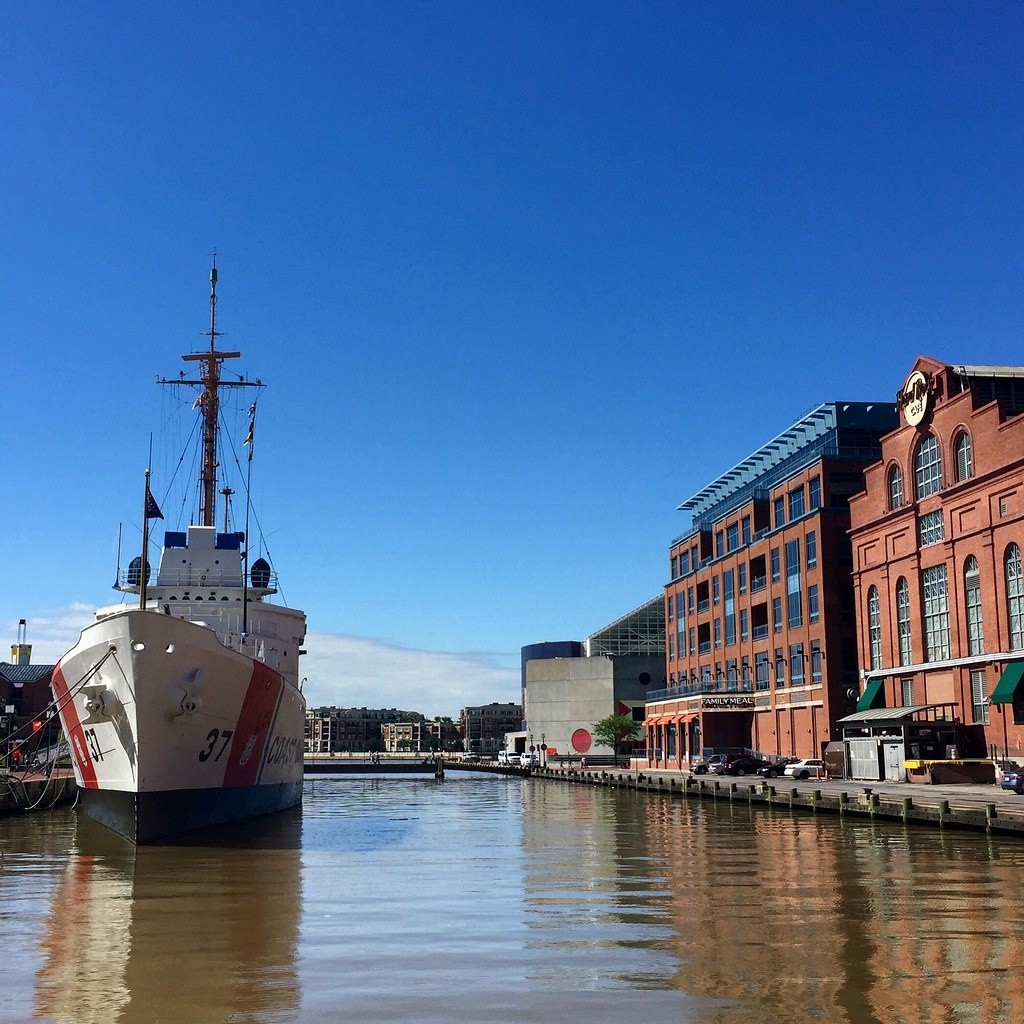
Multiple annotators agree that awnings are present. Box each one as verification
[857,679,885,712]
[991,661,1024,704]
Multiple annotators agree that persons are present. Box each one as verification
[14,749,32,772]
[370,751,436,765]
[560,757,589,770]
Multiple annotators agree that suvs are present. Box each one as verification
[707,753,754,776]
[689,754,722,775]
[519,753,539,768]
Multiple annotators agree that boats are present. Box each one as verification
[47,248,306,846]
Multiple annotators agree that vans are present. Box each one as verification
[497,751,521,766]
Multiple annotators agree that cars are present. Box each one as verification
[1000,765,1024,795]
[783,759,827,780]
[756,759,801,779]
[727,757,775,776]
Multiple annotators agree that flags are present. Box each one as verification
[145,486,165,520]
[244,401,257,462]
[192,391,205,410]
